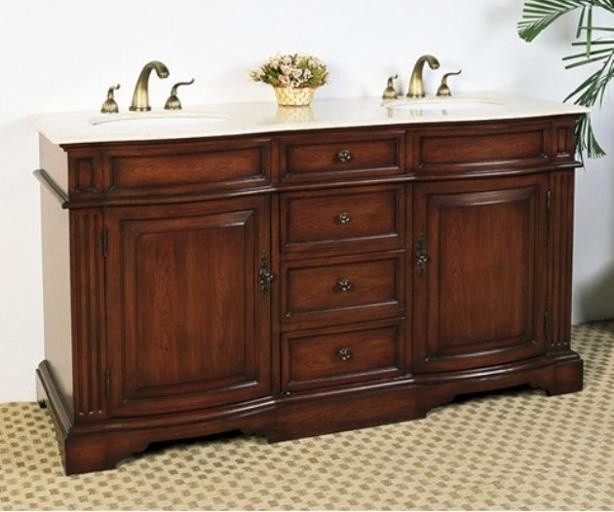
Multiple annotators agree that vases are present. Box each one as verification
[272,82,320,108]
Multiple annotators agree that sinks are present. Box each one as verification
[383,97,505,111]
[91,113,232,132]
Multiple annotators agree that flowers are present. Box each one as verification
[249,52,330,88]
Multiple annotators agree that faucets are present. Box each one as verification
[128,59,170,112]
[406,55,440,98]
